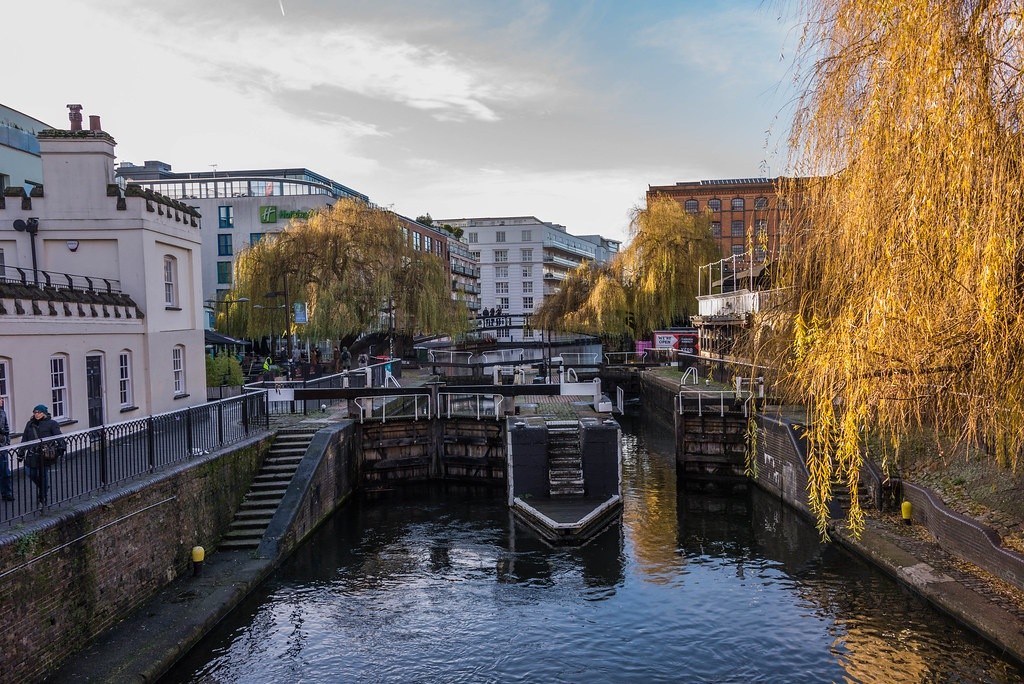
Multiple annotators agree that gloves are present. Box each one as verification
[18,455,22,462]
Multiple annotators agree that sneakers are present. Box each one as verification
[2,494,13,501]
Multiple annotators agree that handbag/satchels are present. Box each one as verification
[32,426,58,459]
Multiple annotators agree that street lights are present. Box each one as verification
[253,303,288,367]
[203,297,249,376]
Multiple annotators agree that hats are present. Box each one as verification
[34,404,48,414]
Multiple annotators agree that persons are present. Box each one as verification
[357,351,368,368]
[311,350,318,364]
[483,306,489,326]
[334,346,340,373]
[263,353,273,371]
[489,308,495,326]
[18,404,67,508]
[340,347,351,370]
[496,307,502,326]
[281,346,287,363]
[316,347,323,363]
[0,396,16,501]
